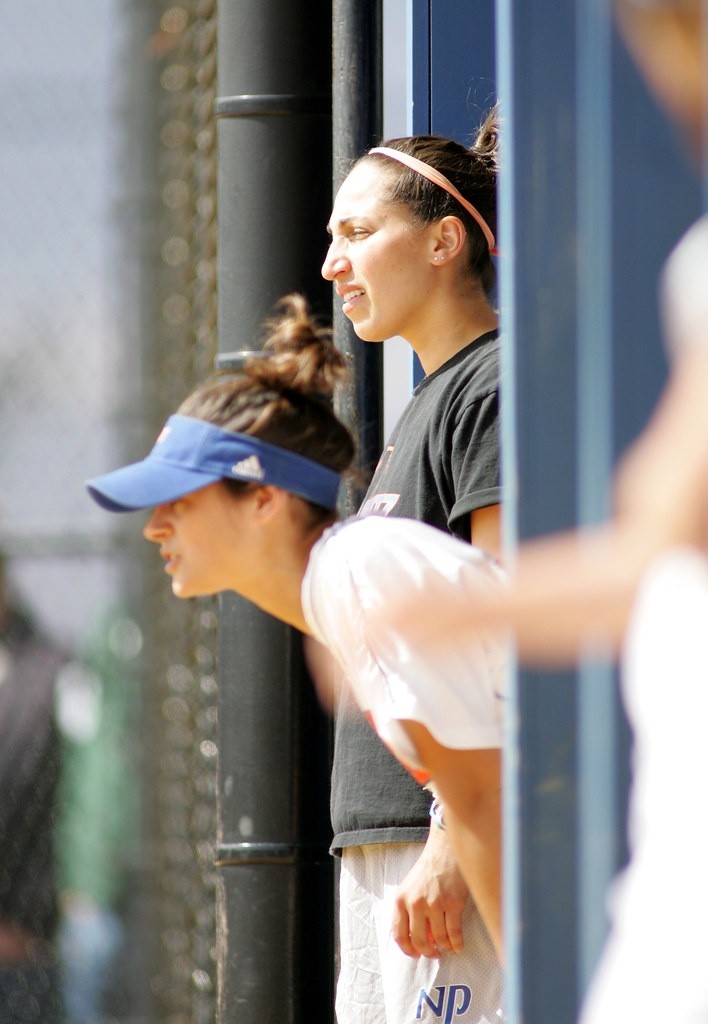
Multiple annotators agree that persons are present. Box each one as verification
[139,294,502,981]
[326,102,502,1024]
[379,0,708,1024]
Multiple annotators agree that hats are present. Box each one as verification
[85,415,341,514]
